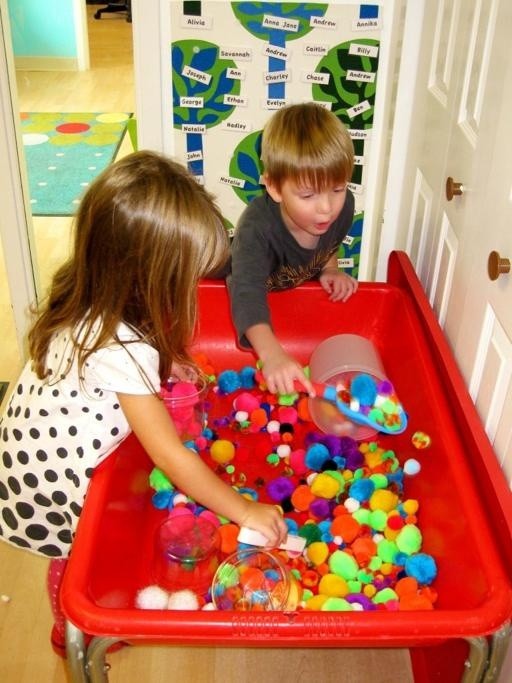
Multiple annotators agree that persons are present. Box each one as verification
[202,101,359,400]
[1,148,289,658]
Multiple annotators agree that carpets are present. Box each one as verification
[19,113,134,216]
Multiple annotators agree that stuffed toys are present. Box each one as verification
[135,360,442,611]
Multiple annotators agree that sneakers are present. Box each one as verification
[51,625,122,658]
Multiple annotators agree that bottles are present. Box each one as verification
[152,515,223,597]
[211,548,299,614]
[161,379,208,443]
